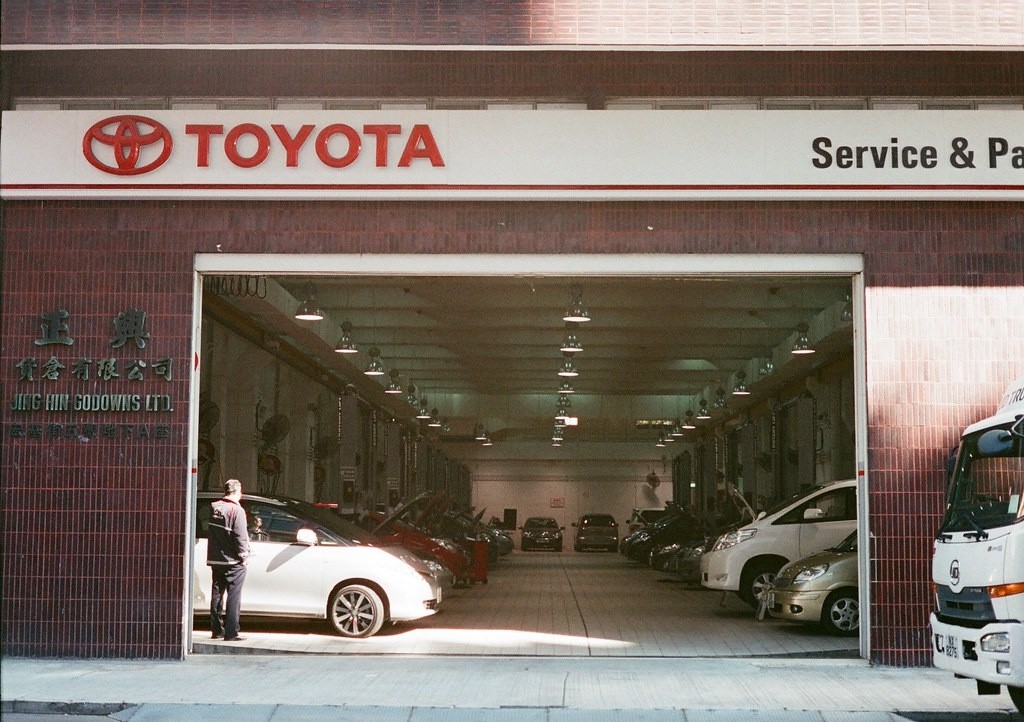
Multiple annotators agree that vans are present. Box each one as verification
[699,480,860,617]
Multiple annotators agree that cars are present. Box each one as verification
[619,507,747,589]
[307,487,515,585]
[192,489,443,639]
[764,529,858,637]
[571,512,619,553]
[518,516,566,552]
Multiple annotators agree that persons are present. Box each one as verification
[247,516,271,542]
[207,479,248,642]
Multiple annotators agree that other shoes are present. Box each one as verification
[211,630,226,639]
[224,635,247,641]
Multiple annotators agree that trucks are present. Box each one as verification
[926,374,1023,720]
